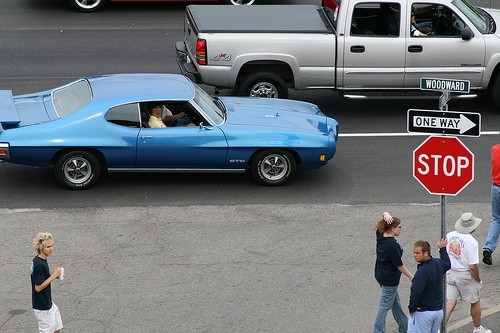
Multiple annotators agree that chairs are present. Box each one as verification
[141,112,151,128]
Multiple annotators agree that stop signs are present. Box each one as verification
[413,136,475,195]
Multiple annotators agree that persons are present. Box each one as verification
[481,143,500,266]
[147,101,168,129]
[29,232,64,333]
[410,10,432,37]
[404,238,452,333]
[372,211,414,333]
[442,212,492,333]
[157,103,192,126]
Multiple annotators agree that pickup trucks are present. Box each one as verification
[176,0,500,108]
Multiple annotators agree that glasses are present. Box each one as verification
[156,105,163,108]
[396,225,401,228]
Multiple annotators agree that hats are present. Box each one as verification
[147,101,165,109]
[455,212,482,234]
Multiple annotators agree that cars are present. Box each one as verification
[0,72,339,188]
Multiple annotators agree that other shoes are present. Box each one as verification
[482,249,492,264]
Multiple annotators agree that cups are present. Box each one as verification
[58,266,65,280]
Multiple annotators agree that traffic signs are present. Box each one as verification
[405,108,483,139]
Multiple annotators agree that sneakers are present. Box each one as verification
[473,325,491,333]
